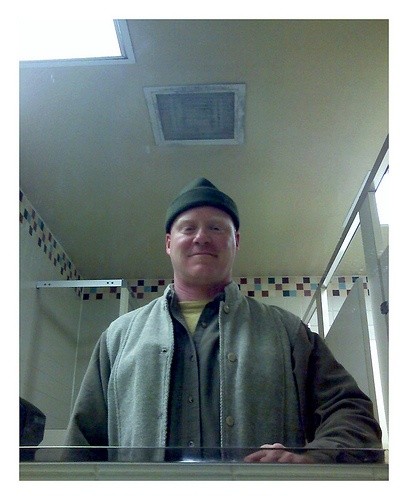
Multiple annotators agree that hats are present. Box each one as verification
[165,177,240,231]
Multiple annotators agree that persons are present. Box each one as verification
[58,176,388,466]
